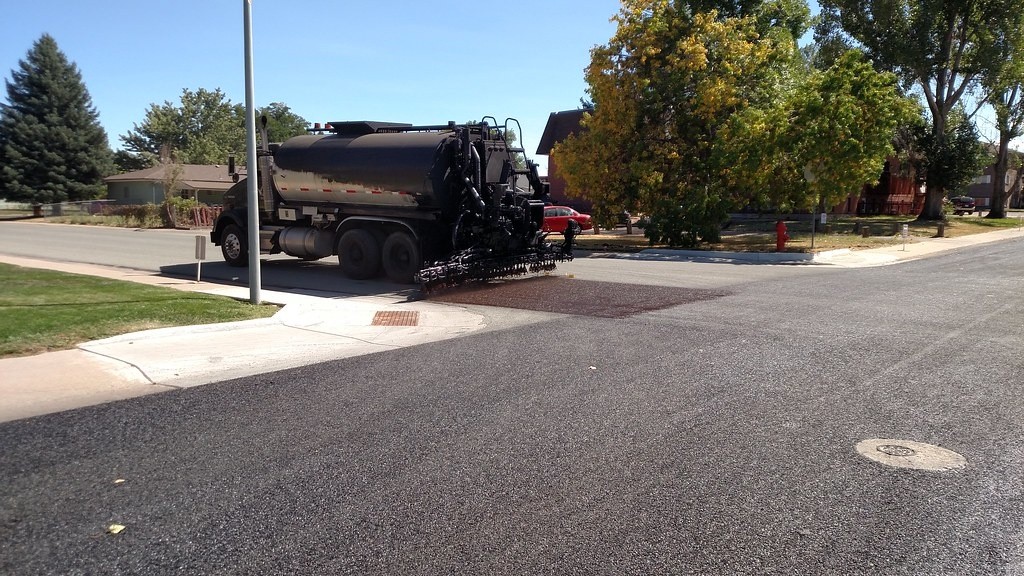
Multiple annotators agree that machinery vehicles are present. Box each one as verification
[210,115,576,293]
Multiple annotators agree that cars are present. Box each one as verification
[541,207,592,235]
[616,211,632,224]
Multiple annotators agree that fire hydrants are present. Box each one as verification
[775,220,790,252]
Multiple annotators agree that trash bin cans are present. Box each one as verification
[31,203,43,217]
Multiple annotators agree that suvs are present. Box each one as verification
[952,197,976,215]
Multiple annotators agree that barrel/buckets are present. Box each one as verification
[279,226,335,256]
